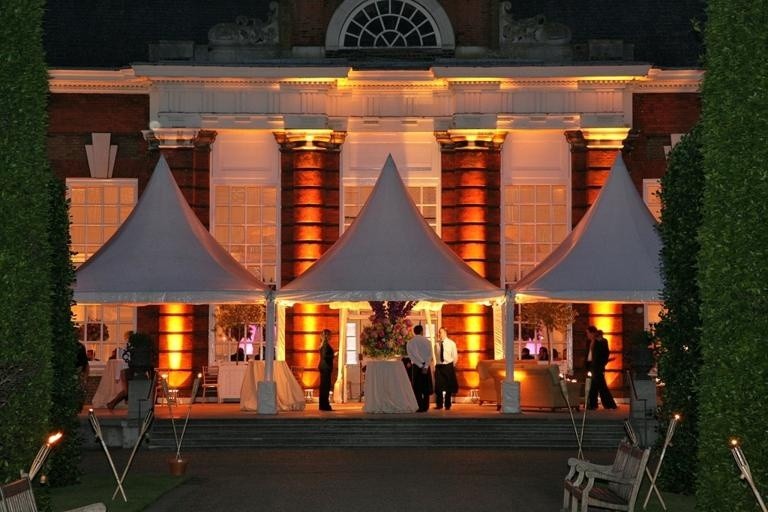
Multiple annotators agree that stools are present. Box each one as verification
[167,388,181,406]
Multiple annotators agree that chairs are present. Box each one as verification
[201,365,220,401]
[153,368,170,404]
[573,448,650,512]
[561,441,633,511]
[1,474,110,511]
[292,365,306,396]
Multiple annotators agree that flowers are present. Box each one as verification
[359,299,411,358]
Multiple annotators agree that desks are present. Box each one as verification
[155,366,200,403]
[217,361,264,408]
[240,357,308,415]
[361,361,424,416]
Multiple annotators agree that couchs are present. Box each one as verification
[476,354,591,413]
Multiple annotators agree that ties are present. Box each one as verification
[440,341,444,363]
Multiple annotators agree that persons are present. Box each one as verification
[107,369,148,411]
[401,357,419,379]
[231,348,248,362]
[516,347,567,360]
[318,328,339,411]
[255,346,276,360]
[406,325,433,412]
[583,326,617,412]
[87,349,99,361]
[108,330,133,359]
[434,327,458,410]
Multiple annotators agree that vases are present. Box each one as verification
[162,454,191,478]
